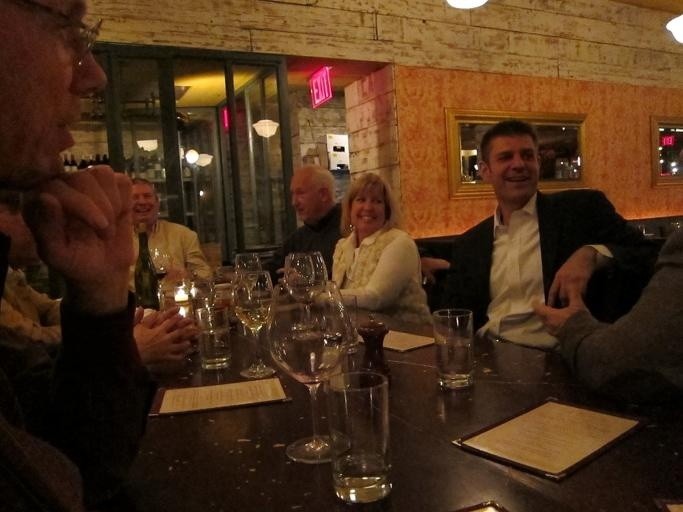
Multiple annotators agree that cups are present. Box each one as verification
[285,254,315,309]
[154,250,169,283]
[284,252,329,342]
[230,271,277,378]
[194,307,232,370]
[433,308,473,389]
[236,253,261,301]
[324,371,392,503]
[268,281,352,465]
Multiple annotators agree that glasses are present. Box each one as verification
[13,0,105,67]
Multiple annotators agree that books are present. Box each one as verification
[453,397,647,481]
[148,377,287,416]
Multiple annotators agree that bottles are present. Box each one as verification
[79,153,88,172]
[88,154,95,170]
[134,222,159,312]
[70,154,78,172]
[62,152,70,172]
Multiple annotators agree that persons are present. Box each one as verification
[0,0,155,511]
[529,224,683,395]
[1,193,194,364]
[312,173,431,323]
[425,119,653,351]
[262,164,450,288]
[128,177,213,309]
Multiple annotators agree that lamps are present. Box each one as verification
[197,154,214,167]
[252,120,279,138]
